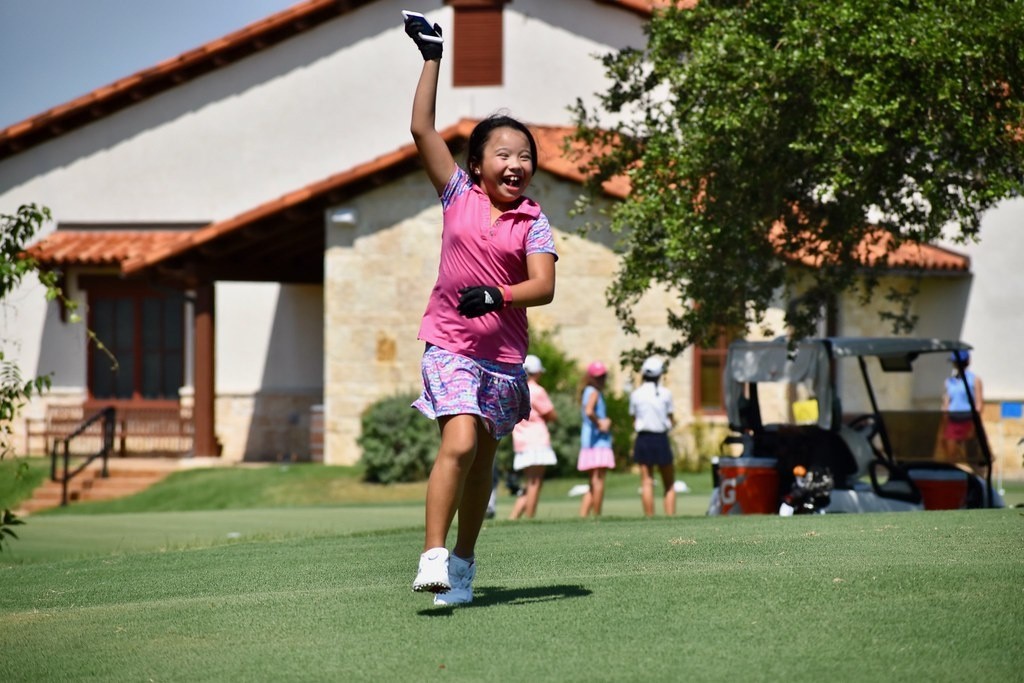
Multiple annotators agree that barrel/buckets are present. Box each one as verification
[716,457,778,516]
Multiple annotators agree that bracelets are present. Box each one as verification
[499,285,513,309]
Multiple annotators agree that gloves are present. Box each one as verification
[455,285,505,318]
[404,17,443,61]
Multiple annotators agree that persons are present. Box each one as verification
[576,362,616,517]
[402,13,558,606]
[629,354,677,516]
[944,349,987,476]
[484,354,558,520]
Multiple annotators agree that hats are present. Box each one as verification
[588,362,606,377]
[523,354,545,373]
[641,358,663,377]
[952,349,969,361]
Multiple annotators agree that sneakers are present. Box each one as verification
[433,553,475,606]
[413,547,452,593]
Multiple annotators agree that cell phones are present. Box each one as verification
[401,10,444,43]
